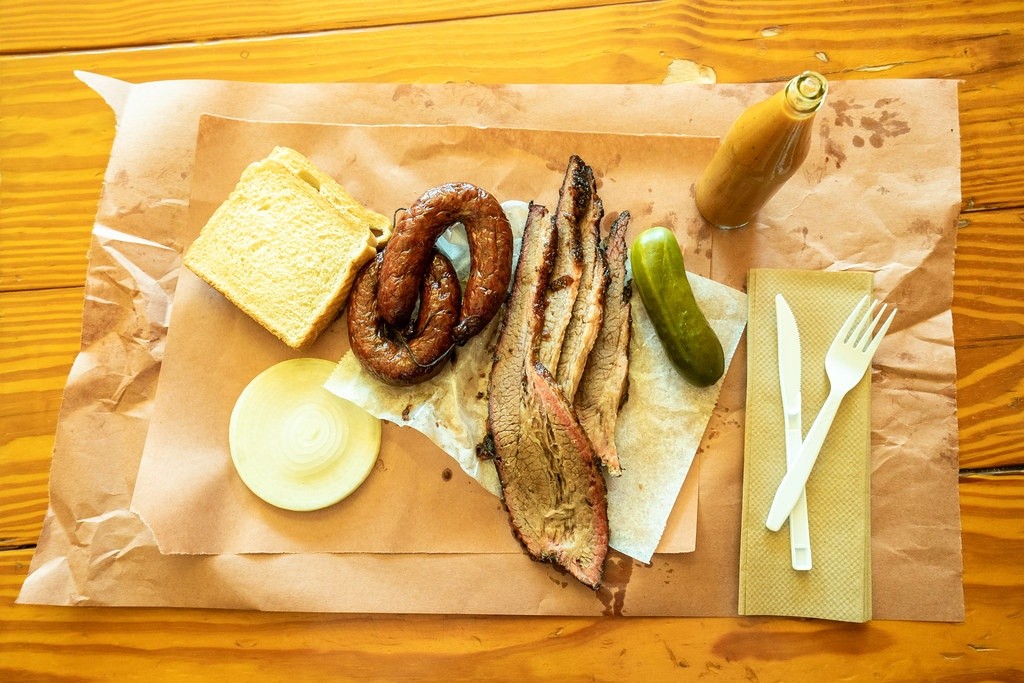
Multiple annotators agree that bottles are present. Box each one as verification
[696,73,826,228]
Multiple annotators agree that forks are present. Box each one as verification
[767,295,897,531]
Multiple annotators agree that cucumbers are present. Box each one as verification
[630,226,725,386]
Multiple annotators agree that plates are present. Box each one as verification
[229,357,380,511]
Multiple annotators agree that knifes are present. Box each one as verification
[775,294,812,571]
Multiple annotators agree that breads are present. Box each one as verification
[185,145,391,352]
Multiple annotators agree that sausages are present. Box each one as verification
[347,244,462,386]
[378,182,514,344]
